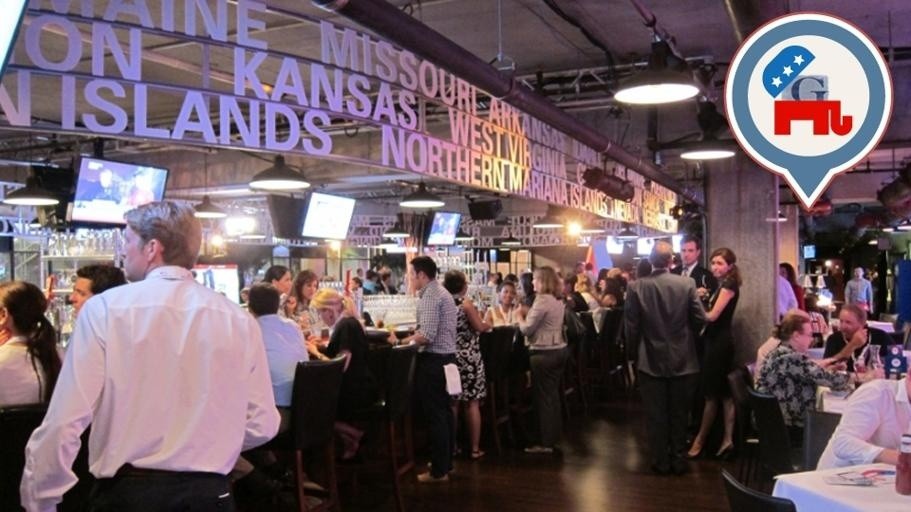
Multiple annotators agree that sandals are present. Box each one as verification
[453,448,462,458]
[470,450,485,460]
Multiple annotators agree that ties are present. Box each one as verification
[683,267,688,277]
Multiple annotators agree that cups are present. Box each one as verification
[362,295,416,325]
[432,256,461,282]
[44,228,119,257]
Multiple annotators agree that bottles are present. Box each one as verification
[895,430,911,493]
[867,343,882,372]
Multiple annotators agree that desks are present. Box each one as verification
[773,463,911,512]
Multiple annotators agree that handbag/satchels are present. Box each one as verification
[514,307,591,364]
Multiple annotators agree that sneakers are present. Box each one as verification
[427,462,459,477]
[417,472,451,483]
[523,443,555,454]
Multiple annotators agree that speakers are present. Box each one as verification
[467,200,503,221]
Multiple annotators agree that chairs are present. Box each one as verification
[337,340,422,512]
[481,327,516,452]
[722,469,796,512]
[745,386,797,473]
[263,358,349,512]
[803,409,841,469]
[560,309,630,414]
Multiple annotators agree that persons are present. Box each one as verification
[229,254,402,512]
[443,269,491,458]
[484,235,743,477]
[20,200,280,512]
[753,263,911,471]
[388,255,457,484]
[0,281,64,410]
[42,264,125,319]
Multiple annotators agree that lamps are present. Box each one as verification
[534,210,561,227]
[584,221,605,235]
[248,155,310,189]
[192,153,227,221]
[1,133,59,209]
[381,239,399,250]
[679,125,736,160]
[398,183,445,208]
[501,236,520,247]
[613,33,699,104]
[617,225,637,240]
[454,229,472,241]
[383,222,407,236]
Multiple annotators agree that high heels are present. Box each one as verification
[713,441,736,461]
[682,441,710,461]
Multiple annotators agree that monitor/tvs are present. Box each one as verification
[298,188,358,242]
[636,237,655,256]
[671,234,685,253]
[424,210,462,247]
[66,154,170,232]
[802,244,816,261]
[266,193,309,240]
[604,237,624,254]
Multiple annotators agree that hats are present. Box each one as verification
[311,288,345,309]
